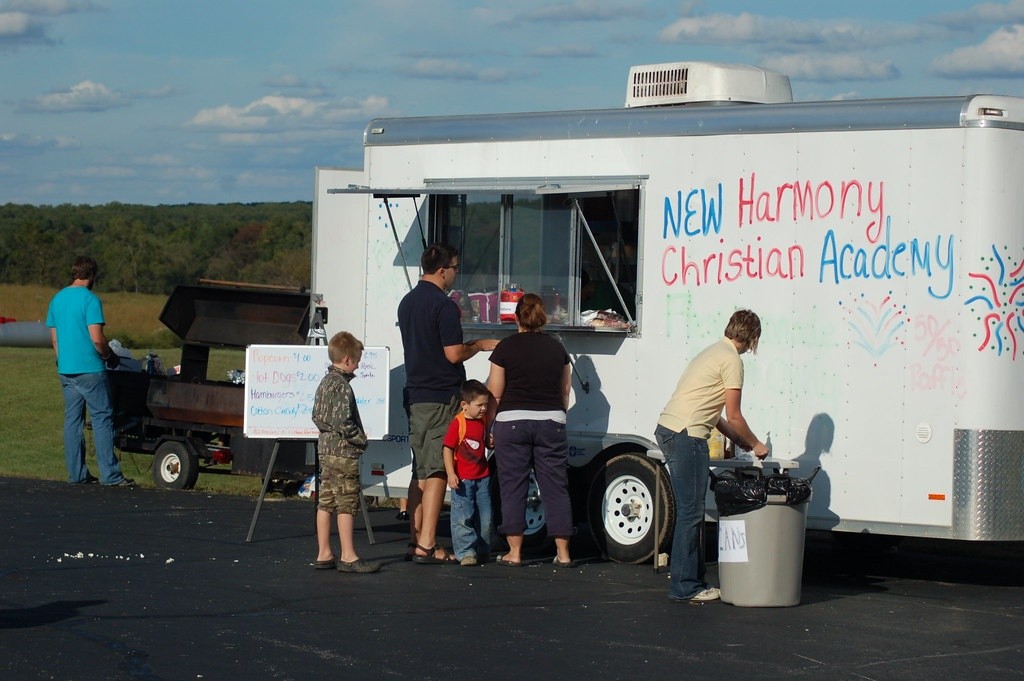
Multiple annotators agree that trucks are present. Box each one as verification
[309,61,1024,565]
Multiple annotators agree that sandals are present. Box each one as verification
[412,543,458,564]
[405,543,415,560]
[338,558,381,573]
[314,555,338,569]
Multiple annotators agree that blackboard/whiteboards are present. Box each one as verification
[243,344,389,440]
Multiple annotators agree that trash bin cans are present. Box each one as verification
[714,467,813,606]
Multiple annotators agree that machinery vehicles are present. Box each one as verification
[105,279,309,489]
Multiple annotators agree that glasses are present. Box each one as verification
[446,264,459,269]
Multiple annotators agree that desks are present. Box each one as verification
[647,450,799,575]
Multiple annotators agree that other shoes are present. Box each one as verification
[113,479,134,486]
[85,476,99,483]
[460,556,478,565]
[691,587,720,601]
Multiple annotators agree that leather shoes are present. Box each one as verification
[396,511,408,520]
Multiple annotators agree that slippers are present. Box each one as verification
[553,556,575,567]
[495,554,522,567]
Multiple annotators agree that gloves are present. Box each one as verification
[102,347,120,369]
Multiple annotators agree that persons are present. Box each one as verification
[45,256,135,487]
[656,311,769,601]
[398,242,501,561]
[442,380,493,567]
[486,293,572,565]
[582,270,635,321]
[311,331,383,572]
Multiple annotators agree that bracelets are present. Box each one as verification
[102,348,112,360]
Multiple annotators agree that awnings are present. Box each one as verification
[327,180,636,318]
